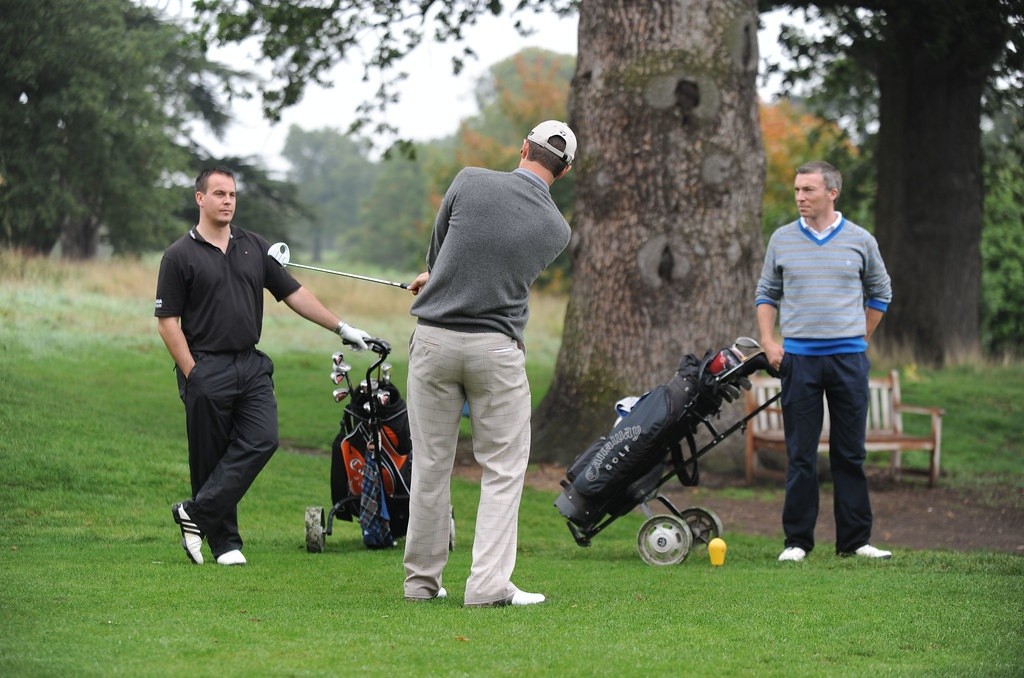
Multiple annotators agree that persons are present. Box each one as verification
[402,119,578,607]
[753,161,893,562]
[153,167,375,565]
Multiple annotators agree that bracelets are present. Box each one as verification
[334,320,345,335]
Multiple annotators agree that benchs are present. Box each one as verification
[745,368,947,493]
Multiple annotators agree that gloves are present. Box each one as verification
[339,323,373,353]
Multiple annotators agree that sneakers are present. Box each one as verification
[216,550,246,566]
[778,547,806,562]
[465,587,545,608]
[405,587,447,602]
[170,501,204,565]
[840,545,893,558]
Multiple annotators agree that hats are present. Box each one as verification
[525,120,579,166]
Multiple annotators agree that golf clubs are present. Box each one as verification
[330,351,393,413]
[267,242,418,291]
[719,337,761,403]
